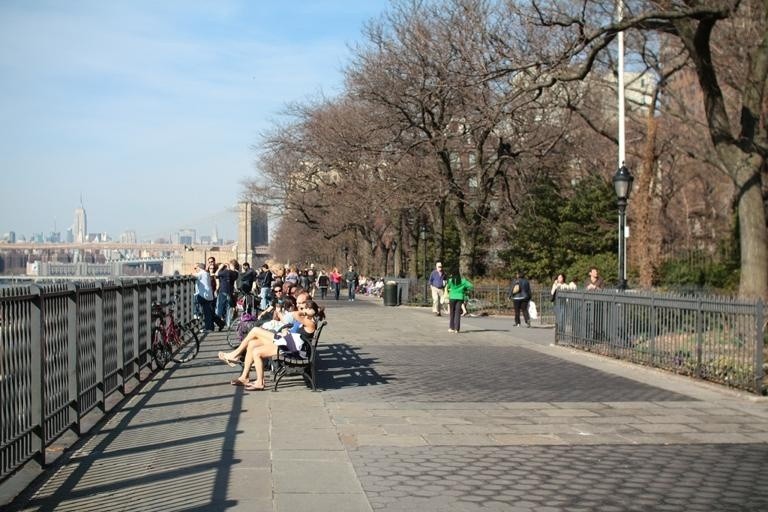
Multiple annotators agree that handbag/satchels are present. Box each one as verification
[227,292,237,309]
[512,279,520,295]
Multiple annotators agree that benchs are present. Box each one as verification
[271,317,326,392]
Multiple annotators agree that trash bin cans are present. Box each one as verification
[384,280,397,306]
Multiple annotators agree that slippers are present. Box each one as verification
[230,378,247,386]
[244,384,264,391]
[218,351,237,368]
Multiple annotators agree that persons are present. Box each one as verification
[582,266,606,291]
[446,269,474,334]
[441,272,468,317]
[568,276,579,290]
[428,260,447,316]
[551,273,570,299]
[191,256,384,392]
[507,269,534,329]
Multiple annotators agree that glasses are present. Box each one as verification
[274,290,281,293]
[300,307,304,311]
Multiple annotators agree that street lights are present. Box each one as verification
[421,223,431,308]
[390,236,399,279]
[610,159,634,289]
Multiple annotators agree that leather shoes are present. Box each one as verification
[219,320,225,332]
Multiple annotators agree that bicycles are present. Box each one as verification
[226,285,262,348]
[148,289,201,374]
